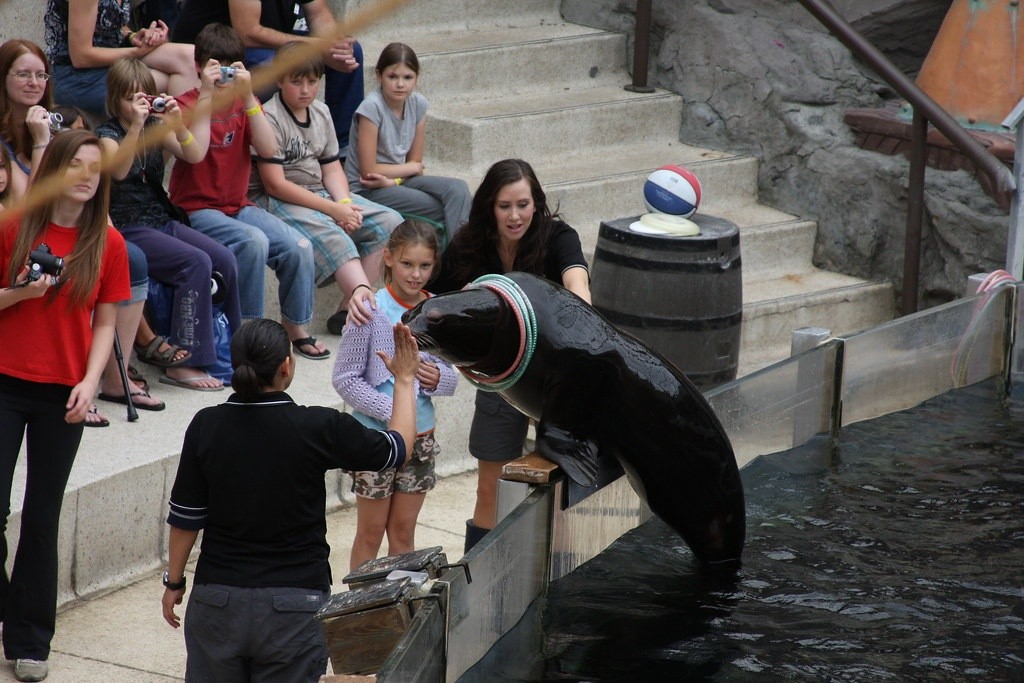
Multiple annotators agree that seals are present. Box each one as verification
[399,269,748,566]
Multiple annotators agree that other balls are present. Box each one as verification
[641,165,700,223]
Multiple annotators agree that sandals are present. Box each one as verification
[125,365,150,392]
[132,336,192,368]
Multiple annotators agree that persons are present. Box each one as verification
[161,318,421,683]
[0,129,133,683]
[0,0,474,427]
[333,217,459,588]
[436,159,590,557]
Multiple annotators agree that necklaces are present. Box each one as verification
[119,121,147,184]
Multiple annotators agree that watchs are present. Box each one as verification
[163,571,187,591]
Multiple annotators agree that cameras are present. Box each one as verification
[44,111,63,132]
[217,66,239,84]
[140,95,166,113]
[28,243,63,280]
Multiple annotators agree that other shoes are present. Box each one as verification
[326,311,347,336]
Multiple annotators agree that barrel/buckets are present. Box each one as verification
[589,212,743,393]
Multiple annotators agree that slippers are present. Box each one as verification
[83,405,110,427]
[159,369,225,392]
[98,388,166,411]
[292,335,331,359]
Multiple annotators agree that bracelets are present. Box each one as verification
[337,197,352,204]
[179,131,194,146]
[395,178,403,186]
[350,283,373,298]
[125,31,131,43]
[243,101,261,116]
[32,143,47,149]
[128,33,136,43]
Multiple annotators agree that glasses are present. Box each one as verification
[9,72,51,82]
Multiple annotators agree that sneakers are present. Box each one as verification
[13,658,48,682]
